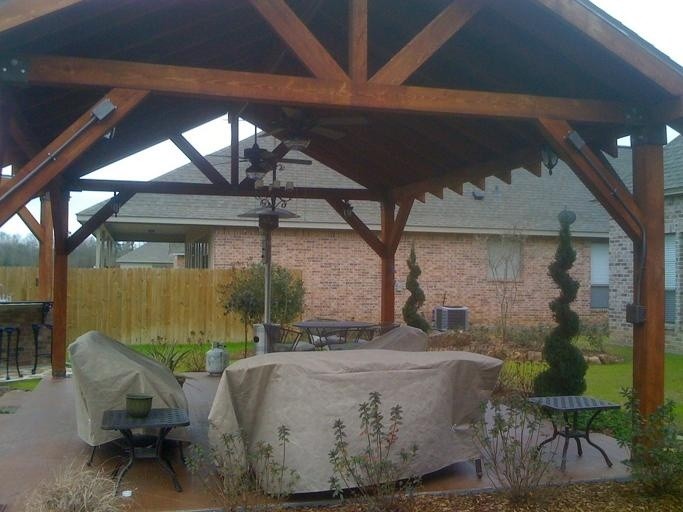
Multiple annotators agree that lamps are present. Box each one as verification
[245,161,268,180]
[253,163,294,208]
[540,141,558,176]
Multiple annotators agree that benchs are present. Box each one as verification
[0,301,53,379]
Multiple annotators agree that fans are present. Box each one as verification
[207,123,312,166]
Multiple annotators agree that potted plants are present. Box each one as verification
[149,335,193,388]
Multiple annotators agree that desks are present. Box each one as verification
[525,395,622,472]
[291,322,379,345]
[100,408,190,497]
